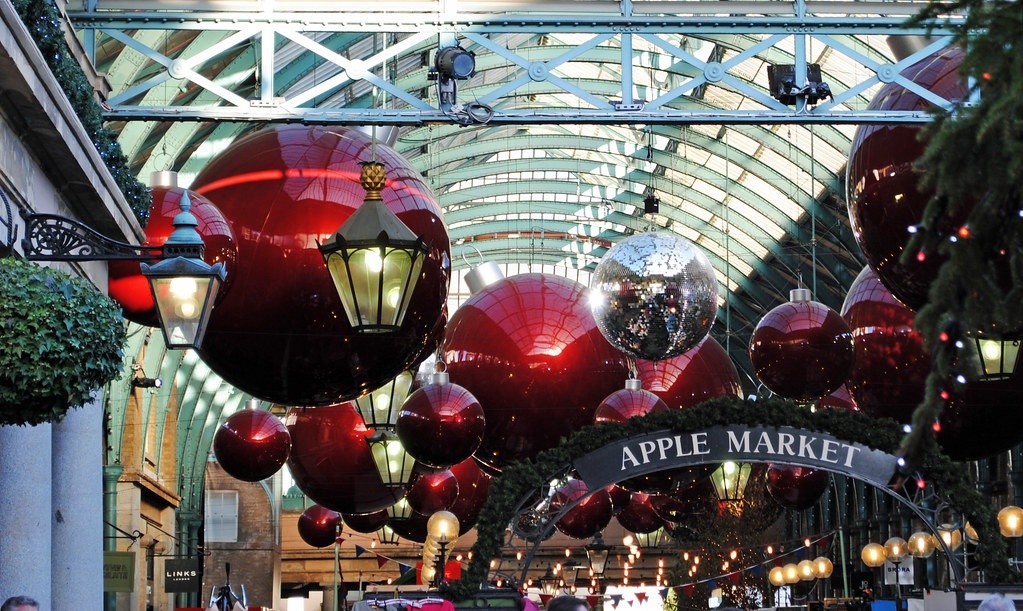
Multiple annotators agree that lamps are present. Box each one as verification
[537,561,561,598]
[636,526,664,549]
[363,431,417,488]
[709,461,753,503]
[768,556,833,606]
[377,525,400,546]
[862,505,1023,599]
[356,366,418,431]
[962,331,1023,383]
[584,532,616,577]
[420,510,460,588]
[316,32,434,334]
[562,557,582,587]
[0,181,230,352]
[386,497,413,521]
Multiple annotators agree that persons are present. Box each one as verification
[548,596,588,611]
[978,596,1016,611]
[1,596,39,611]
